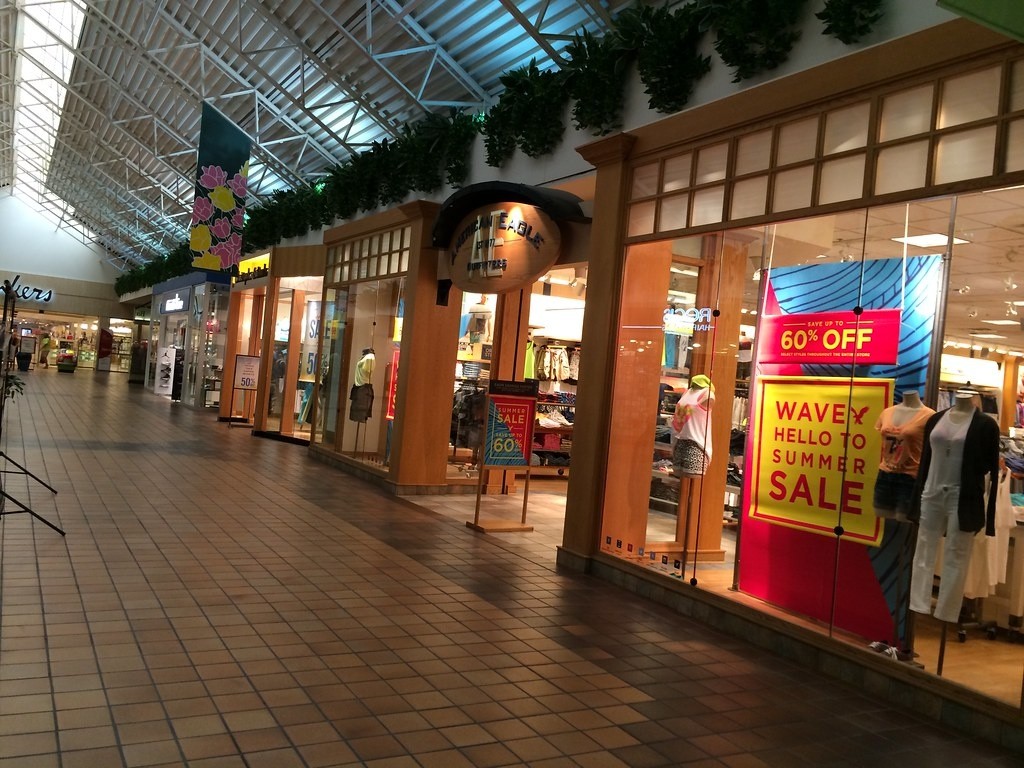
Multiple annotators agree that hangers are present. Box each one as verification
[527,339,537,347]
[999,437,1024,480]
[542,341,567,350]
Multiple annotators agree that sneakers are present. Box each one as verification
[161,367,172,372]
[869,641,912,662]
[161,373,170,379]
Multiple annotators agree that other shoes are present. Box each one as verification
[162,361,171,366]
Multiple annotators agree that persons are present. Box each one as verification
[38,333,51,370]
[909,395,1001,626]
[670,373,715,480]
[872,390,938,527]
[349,349,376,425]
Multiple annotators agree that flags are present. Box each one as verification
[187,99,251,277]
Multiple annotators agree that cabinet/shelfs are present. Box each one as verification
[531,403,575,476]
[77,343,95,368]
[722,448,741,528]
[204,370,222,409]
[648,391,680,516]
